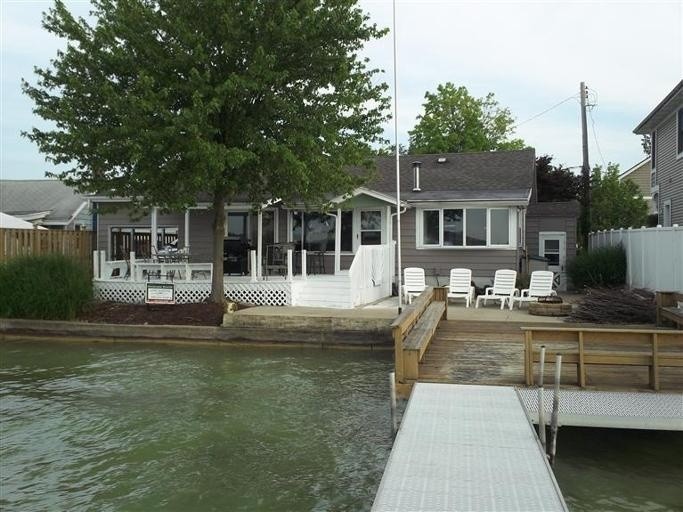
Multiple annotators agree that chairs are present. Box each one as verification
[401,267,429,305]
[120,244,205,280]
[443,268,557,310]
[241,243,324,276]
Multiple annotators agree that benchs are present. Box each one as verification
[660,305,683,329]
[524,349,682,391]
[402,300,445,380]
